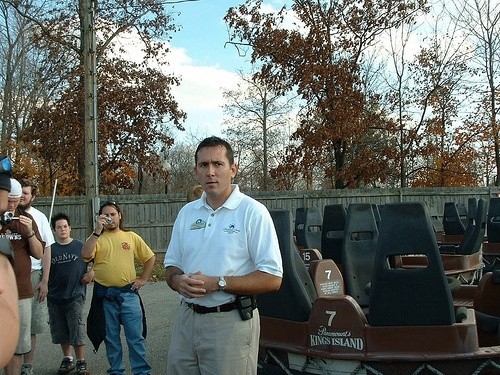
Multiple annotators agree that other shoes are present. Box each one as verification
[21,363,34,375]
[76,360,90,375]
[57,357,75,375]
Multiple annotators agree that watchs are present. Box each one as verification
[218,276,226,293]
[26,230,36,238]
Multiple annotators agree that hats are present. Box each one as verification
[7,178,23,198]
[0,172,11,193]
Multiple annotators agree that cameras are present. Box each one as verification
[3,211,19,221]
[104,216,112,224]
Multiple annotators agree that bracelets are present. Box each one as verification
[93,231,100,239]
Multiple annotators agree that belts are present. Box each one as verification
[185,296,258,314]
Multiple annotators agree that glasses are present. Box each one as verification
[109,201,118,207]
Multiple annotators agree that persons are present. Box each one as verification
[42,213,94,375]
[82,203,155,375]
[163,137,283,375]
[0,153,55,375]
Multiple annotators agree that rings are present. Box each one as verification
[27,221,29,223]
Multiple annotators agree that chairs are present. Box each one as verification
[257,197,500,326]
